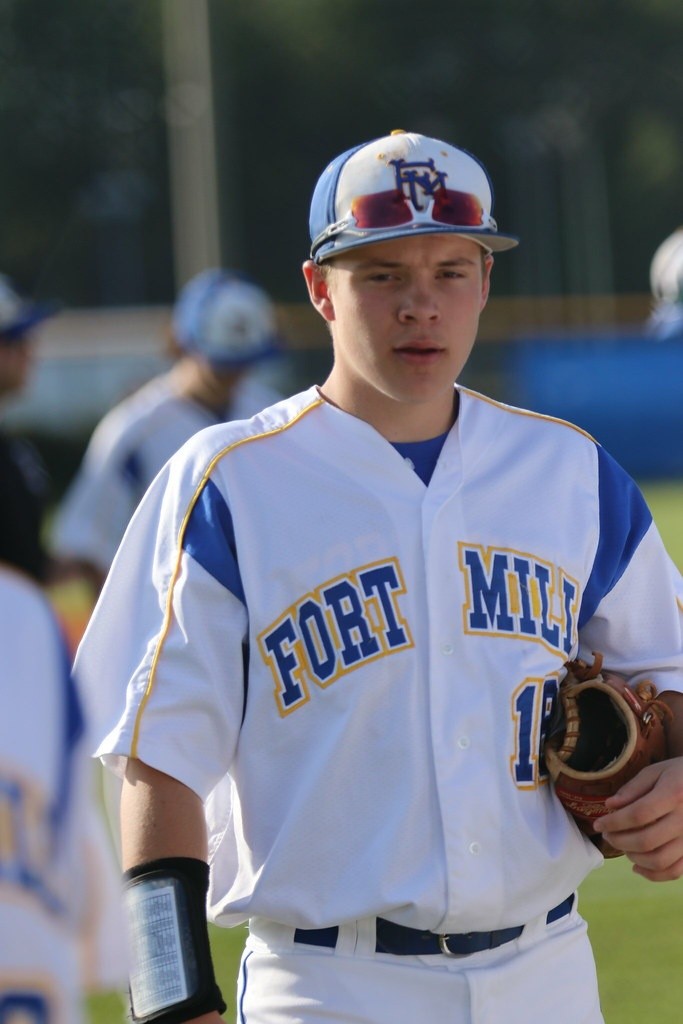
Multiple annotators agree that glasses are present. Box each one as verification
[311,187,498,257]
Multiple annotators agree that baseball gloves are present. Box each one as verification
[546,650,668,822]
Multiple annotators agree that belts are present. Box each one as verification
[292,891,577,959]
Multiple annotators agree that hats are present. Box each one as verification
[169,267,285,374]
[645,228,683,341]
[307,128,523,264]
[0,271,62,340]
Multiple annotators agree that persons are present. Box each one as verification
[1,560,127,1023]
[43,268,288,606]
[91,131,683,1023]
[1,277,59,585]
